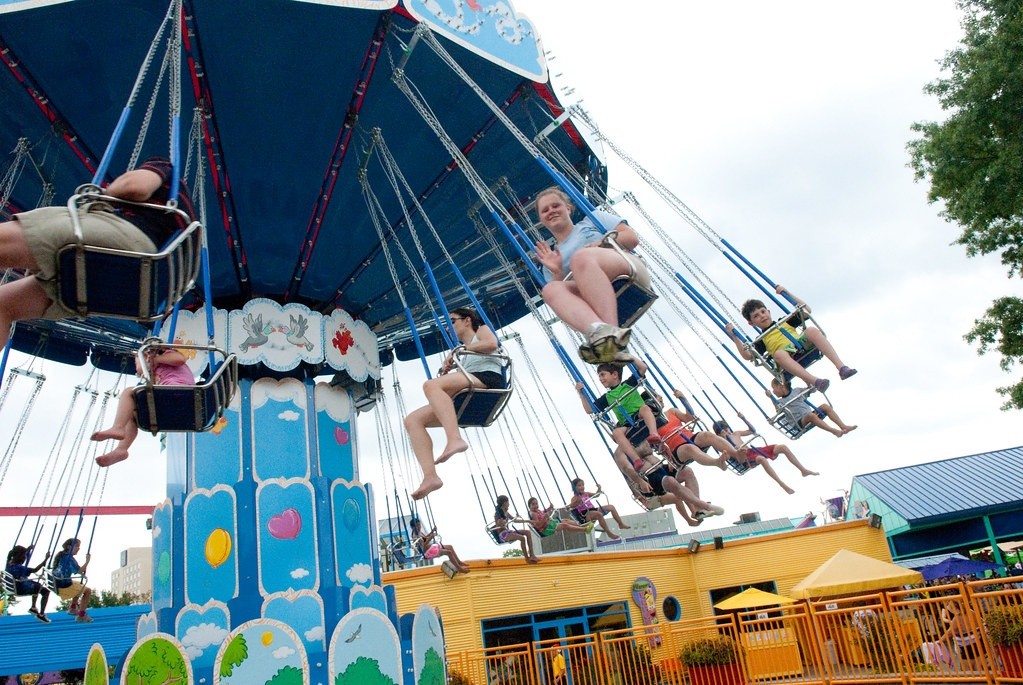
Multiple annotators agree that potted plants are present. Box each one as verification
[842,611,908,675]
[981,603,1023,677]
[680,636,747,685]
[629,645,661,685]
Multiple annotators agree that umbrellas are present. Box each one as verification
[712,586,799,611]
[920,557,1004,580]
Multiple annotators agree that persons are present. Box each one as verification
[726,284,858,391]
[533,188,651,366]
[571,479,631,539]
[997,583,1022,607]
[53,538,94,623]
[851,601,879,668]
[403,308,505,500]
[641,390,746,471]
[1008,560,1023,588]
[936,598,985,671]
[614,444,725,525]
[551,644,566,685]
[410,518,470,573]
[712,411,821,494]
[574,354,660,471]
[0,156,200,352]
[6,545,51,623]
[528,497,594,536]
[498,651,518,685]
[765,378,858,438]
[91,339,196,469]
[494,495,541,564]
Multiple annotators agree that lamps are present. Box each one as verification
[714,536,724,549]
[688,538,701,553]
[868,513,882,529]
[442,560,458,579]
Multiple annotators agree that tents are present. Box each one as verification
[791,548,926,600]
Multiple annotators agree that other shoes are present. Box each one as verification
[708,504,725,515]
[697,509,715,518]
[68,605,79,614]
[838,365,858,380]
[36,614,51,624]
[28,608,39,615]
[815,379,829,392]
[585,523,594,534]
[589,324,633,347]
[75,613,94,622]
[579,347,634,365]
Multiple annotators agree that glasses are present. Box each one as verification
[450,317,466,324]
